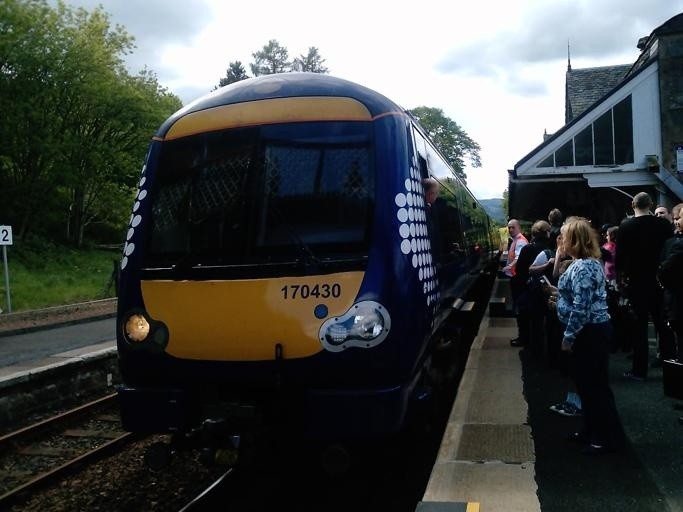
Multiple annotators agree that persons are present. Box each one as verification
[500,191,683,448]
[423,178,440,242]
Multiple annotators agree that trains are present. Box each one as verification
[117,71,503,472]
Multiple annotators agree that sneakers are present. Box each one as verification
[550,402,583,416]
